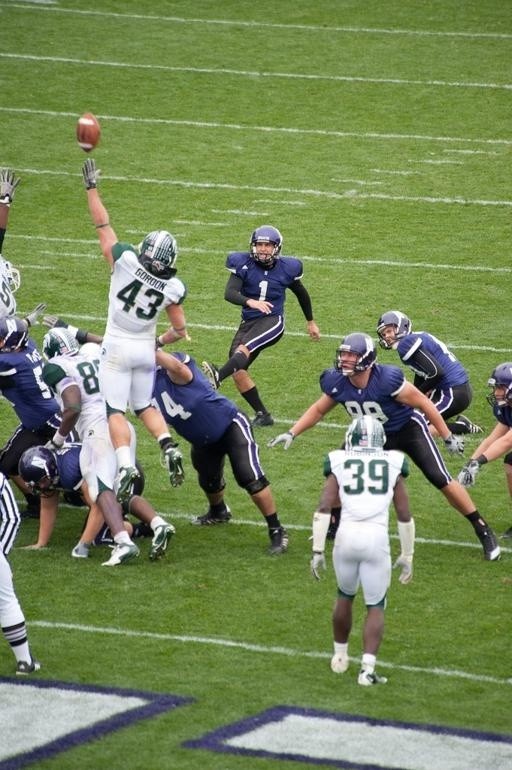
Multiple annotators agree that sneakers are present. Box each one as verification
[201,360,222,392]
[268,524,289,555]
[160,443,184,487]
[102,520,175,566]
[330,651,349,673]
[455,413,484,434]
[188,503,232,525]
[357,669,388,687]
[114,465,141,505]
[251,411,274,427]
[478,528,500,560]
[16,658,42,676]
[21,507,40,519]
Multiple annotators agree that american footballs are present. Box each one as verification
[78,111,100,152]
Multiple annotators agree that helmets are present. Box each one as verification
[135,230,178,276]
[334,332,378,378]
[18,446,59,497]
[0,316,30,352]
[485,361,512,409]
[42,327,81,361]
[377,310,411,352]
[249,224,283,270]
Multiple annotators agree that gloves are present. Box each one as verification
[44,438,65,451]
[310,551,328,582]
[458,458,480,489]
[83,156,103,190]
[392,554,414,585]
[443,431,467,462]
[267,429,294,451]
[71,541,92,558]
[16,545,46,551]
[0,167,22,208]
[24,303,67,328]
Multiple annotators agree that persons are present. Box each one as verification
[377,311,486,437]
[200,225,321,428]
[310,415,415,686]
[18,317,290,570]
[267,333,500,561]
[0,471,41,676]
[457,361,512,539]
[81,161,186,503]
[1,168,61,480]
